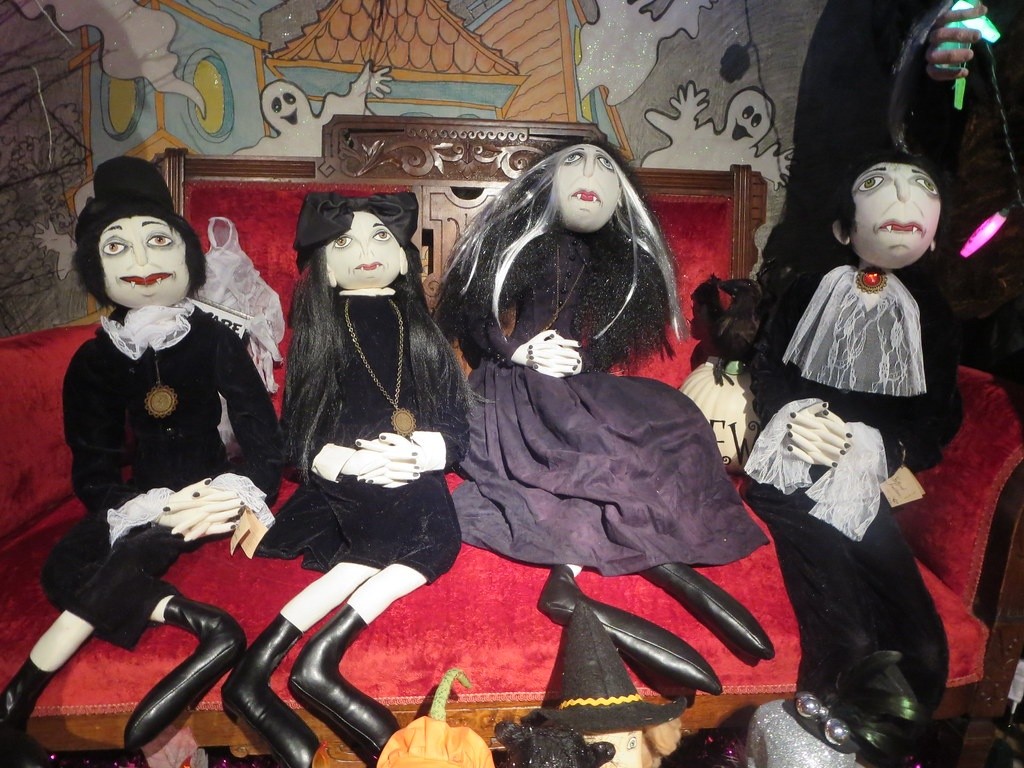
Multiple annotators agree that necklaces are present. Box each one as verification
[344,296,417,437]
[541,240,586,330]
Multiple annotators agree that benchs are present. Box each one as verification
[0,113,1024,768]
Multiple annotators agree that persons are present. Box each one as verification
[219,191,476,768]
[741,156,965,714]
[431,138,774,695]
[728,1,1024,375]
[0,155,285,763]
[494,599,687,768]
